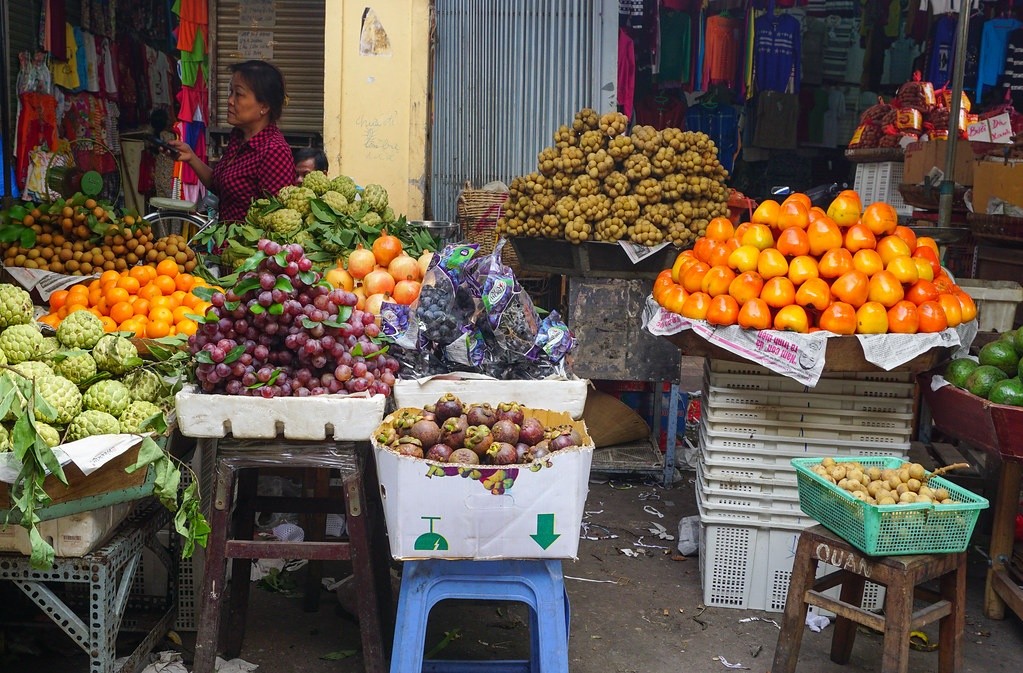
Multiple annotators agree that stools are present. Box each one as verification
[390,560,571,673]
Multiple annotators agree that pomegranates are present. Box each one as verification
[325,228,436,318]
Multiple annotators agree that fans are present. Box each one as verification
[45,138,122,207]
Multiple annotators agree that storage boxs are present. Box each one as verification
[394,379,587,420]
[971,160,1023,214]
[902,141,974,186]
[854,162,913,216]
[694,360,915,619]
[954,278,1023,333]
[371,407,596,561]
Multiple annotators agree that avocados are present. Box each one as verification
[941,327,1023,408]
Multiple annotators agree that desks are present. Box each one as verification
[522,263,683,486]
[984,457,1023,621]
[193,441,388,673]
[771,525,967,673]
[0,496,179,673]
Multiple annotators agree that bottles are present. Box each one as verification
[684,394,700,449]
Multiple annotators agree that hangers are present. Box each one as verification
[702,91,719,108]
[654,88,668,105]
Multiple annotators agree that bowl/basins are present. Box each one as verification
[407,220,458,238]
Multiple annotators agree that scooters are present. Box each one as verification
[769,182,849,216]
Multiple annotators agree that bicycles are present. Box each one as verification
[139,192,225,278]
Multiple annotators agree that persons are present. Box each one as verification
[293,147,329,186]
[143,108,181,237]
[158,59,297,275]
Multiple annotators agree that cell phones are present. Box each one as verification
[148,134,178,153]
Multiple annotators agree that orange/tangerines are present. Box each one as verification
[38,258,225,339]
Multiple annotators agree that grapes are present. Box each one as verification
[184,239,401,397]
[384,259,576,379]
